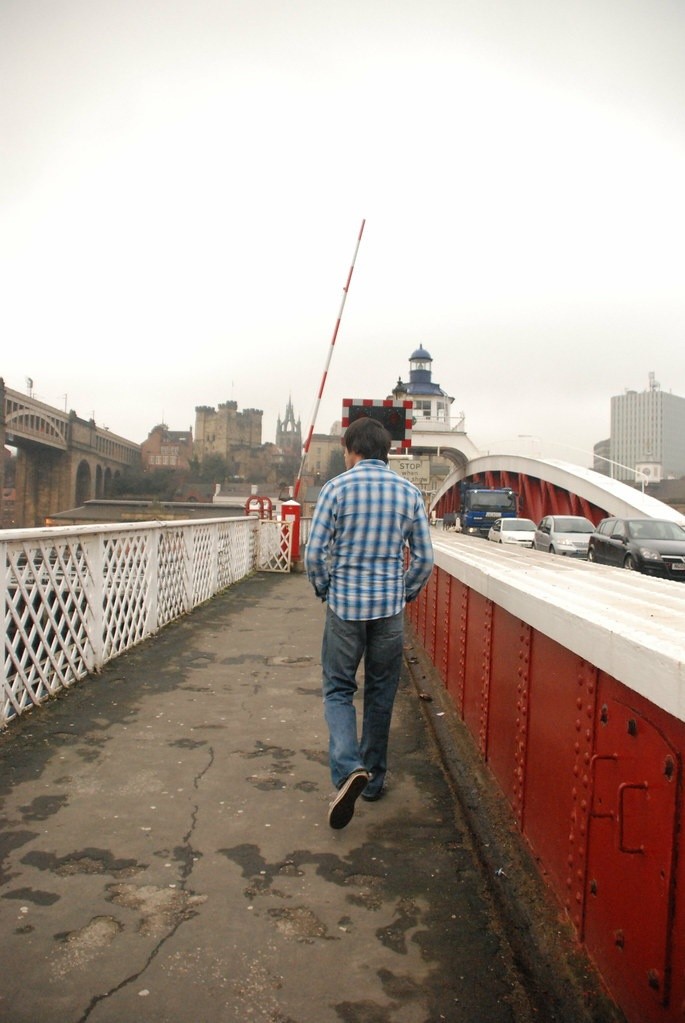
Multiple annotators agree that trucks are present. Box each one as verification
[459,482,520,536]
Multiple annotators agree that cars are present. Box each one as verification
[530,514,597,560]
[485,517,538,548]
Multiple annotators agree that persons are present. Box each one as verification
[305,417,434,832]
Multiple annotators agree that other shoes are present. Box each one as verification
[362,794,380,802]
[327,771,369,830]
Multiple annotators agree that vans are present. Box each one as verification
[587,517,685,581]
